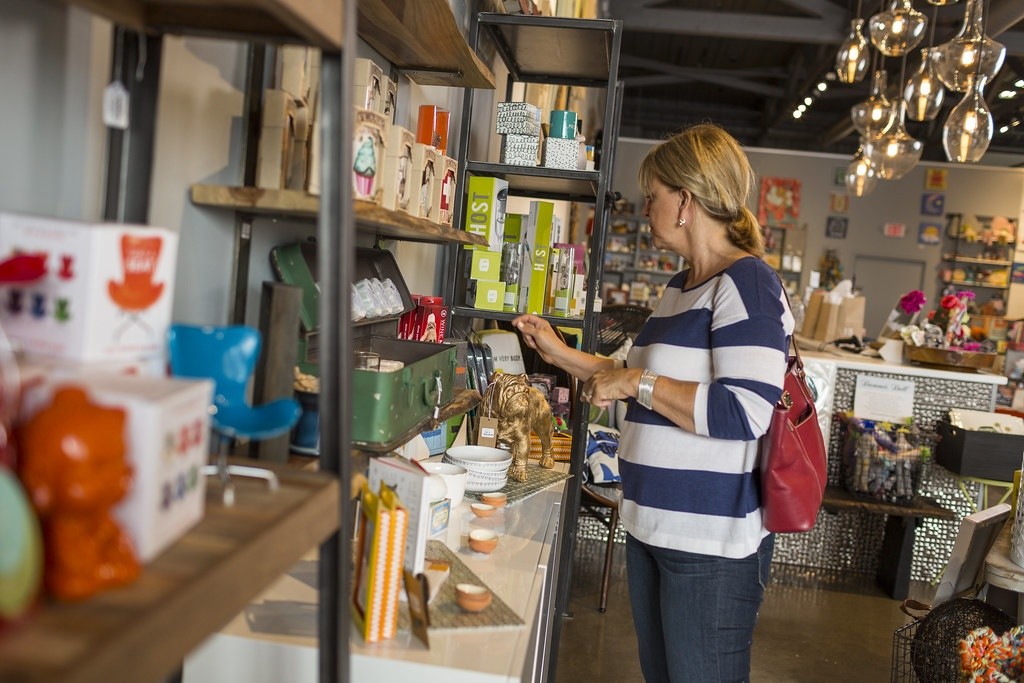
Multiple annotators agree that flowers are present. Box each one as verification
[881,290,928,343]
[941,291,976,346]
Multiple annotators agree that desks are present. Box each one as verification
[930,475,1014,586]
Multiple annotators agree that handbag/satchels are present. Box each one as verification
[758,283,829,533]
[801,289,865,346]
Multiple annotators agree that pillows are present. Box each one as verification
[585,423,621,483]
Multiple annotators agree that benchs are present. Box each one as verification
[822,484,956,601]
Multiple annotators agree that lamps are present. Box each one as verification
[836,0,1007,197]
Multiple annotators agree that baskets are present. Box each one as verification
[959,625,1023,683]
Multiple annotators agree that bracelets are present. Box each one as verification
[636,368,658,410]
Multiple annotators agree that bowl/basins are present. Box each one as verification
[419,445,514,611]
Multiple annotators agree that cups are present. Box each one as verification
[549,110,578,139]
[501,239,575,319]
[352,350,380,373]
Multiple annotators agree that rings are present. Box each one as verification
[583,392,592,398]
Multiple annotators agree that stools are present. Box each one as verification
[579,483,623,613]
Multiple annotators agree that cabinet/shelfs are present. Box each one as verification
[191,0,481,459]
[602,213,787,310]
[184,449,571,683]
[445,11,625,619]
[943,213,1019,317]
[0,0,353,683]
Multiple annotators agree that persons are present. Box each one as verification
[511,123,795,683]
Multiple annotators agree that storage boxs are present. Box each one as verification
[935,420,1024,483]
[0,213,216,565]
[270,238,457,452]
[463,99,586,403]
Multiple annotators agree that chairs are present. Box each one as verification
[168,322,302,507]
